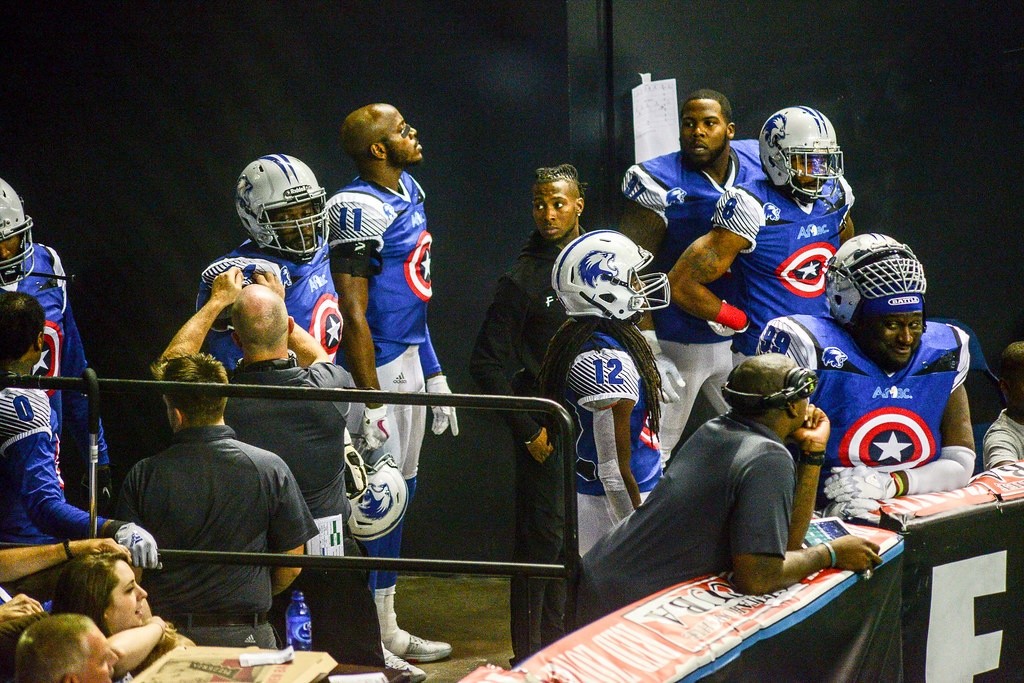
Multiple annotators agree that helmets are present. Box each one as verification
[346,440,408,540]
[235,154,330,264]
[552,231,670,325]
[759,106,844,204]
[0,178,34,286]
[825,233,927,333]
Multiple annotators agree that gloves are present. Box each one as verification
[363,404,389,451]
[640,330,686,404]
[103,520,162,570]
[427,376,459,436]
[817,466,896,522]
[707,300,749,336]
[344,445,368,500]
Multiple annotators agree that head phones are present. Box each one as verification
[720,364,818,413]
[236,350,298,374]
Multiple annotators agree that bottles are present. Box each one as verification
[285,591,312,652]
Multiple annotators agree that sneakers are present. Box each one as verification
[382,629,452,664]
[381,641,427,683]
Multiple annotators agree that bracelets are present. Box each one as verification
[894,472,904,497]
[62,538,74,560]
[894,470,909,496]
[821,541,836,569]
[890,472,900,498]
[797,446,825,466]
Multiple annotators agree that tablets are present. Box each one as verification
[801,516,853,550]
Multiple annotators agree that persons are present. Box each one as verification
[1,102,458,683]
[469,88,1024,666]
